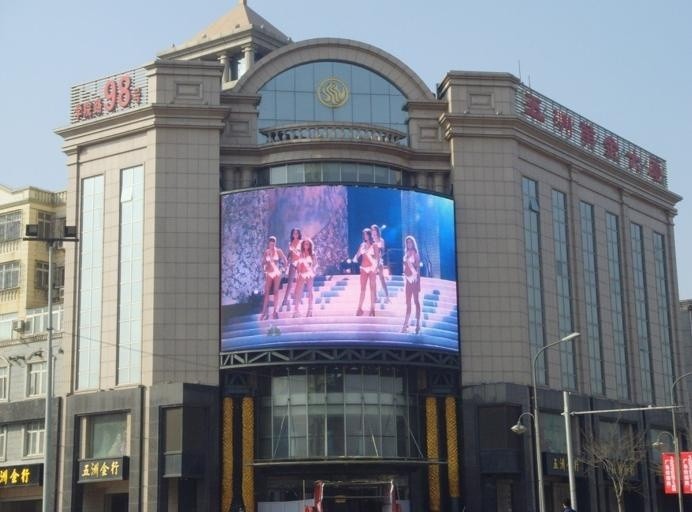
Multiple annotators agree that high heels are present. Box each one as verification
[356,310,376,316]
[262,312,279,320]
[401,326,421,334]
[293,311,313,318]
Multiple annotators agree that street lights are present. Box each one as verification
[652,373,691,512]
[510,331,581,512]
[19,223,85,512]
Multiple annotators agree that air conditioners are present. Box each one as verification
[13,320,24,330]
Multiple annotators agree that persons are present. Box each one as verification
[369,224,392,304]
[400,236,422,334]
[278,227,306,305]
[290,239,320,317]
[258,235,289,320]
[351,227,381,316]
